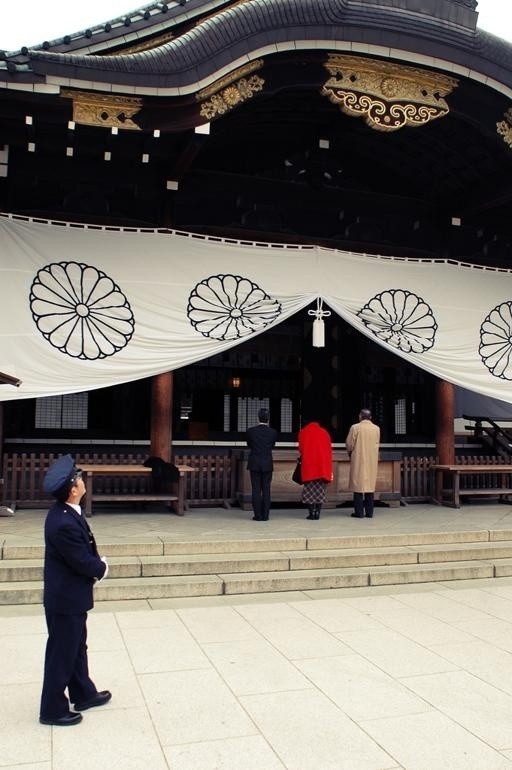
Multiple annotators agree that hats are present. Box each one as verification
[42,454,84,495]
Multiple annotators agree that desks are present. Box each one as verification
[76,462,197,517]
[431,464,512,509]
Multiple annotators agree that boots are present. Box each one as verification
[306,503,314,520]
[314,503,321,520]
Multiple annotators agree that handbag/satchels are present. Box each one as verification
[291,456,305,486]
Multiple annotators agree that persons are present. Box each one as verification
[39,455,112,725]
[298,415,334,522]
[245,408,277,521]
[345,409,380,518]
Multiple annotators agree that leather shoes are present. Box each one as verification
[252,516,262,521]
[351,513,364,518]
[73,690,112,711]
[39,712,83,726]
[364,513,373,518]
[262,516,269,521]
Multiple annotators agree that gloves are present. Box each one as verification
[93,556,110,582]
